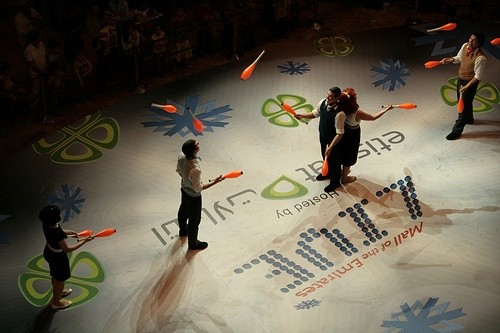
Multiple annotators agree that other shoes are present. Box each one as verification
[324,183,341,192]
[446,131,461,140]
[341,176,356,183]
[455,119,475,125]
[316,173,331,180]
[189,242,208,250]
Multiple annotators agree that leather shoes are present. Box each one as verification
[51,301,72,309]
[61,288,72,296]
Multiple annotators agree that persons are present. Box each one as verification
[441,32,488,140]
[39,204,95,309]
[294,87,348,192]
[338,87,395,183]
[0,0,323,115]
[175,139,226,250]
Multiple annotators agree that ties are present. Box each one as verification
[325,104,328,109]
